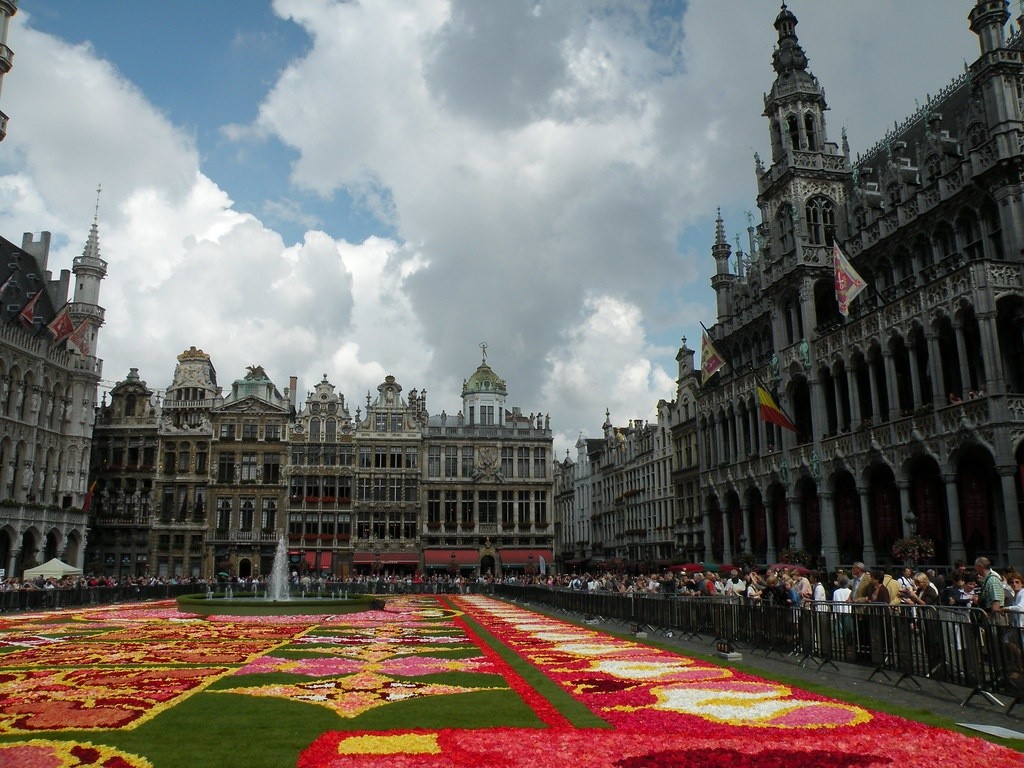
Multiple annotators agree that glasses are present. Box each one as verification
[766,571,771,574]
[1010,580,1022,585]
[838,571,843,574]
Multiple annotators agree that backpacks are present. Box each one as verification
[1000,574,1016,606]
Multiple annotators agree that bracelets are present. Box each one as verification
[1003,606,1008,612]
[913,595,919,602]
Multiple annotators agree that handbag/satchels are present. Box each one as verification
[574,579,582,589]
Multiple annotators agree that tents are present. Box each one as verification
[24,558,82,582]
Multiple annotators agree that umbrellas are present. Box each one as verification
[218,572,229,576]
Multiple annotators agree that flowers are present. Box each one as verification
[892,534,936,565]
[777,546,812,566]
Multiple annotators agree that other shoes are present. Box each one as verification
[1011,672,1021,679]
[25,606,34,613]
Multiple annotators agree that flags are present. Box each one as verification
[83,463,104,513]
[834,239,869,317]
[67,316,89,359]
[756,375,797,432]
[701,329,726,385]
[48,304,75,352]
[17,289,43,330]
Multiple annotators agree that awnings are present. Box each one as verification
[353,553,421,564]
[498,550,556,568]
[423,550,481,568]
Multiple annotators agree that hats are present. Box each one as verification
[837,575,849,587]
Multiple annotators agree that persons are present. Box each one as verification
[768,382,1014,453]
[0,558,1024,680]
